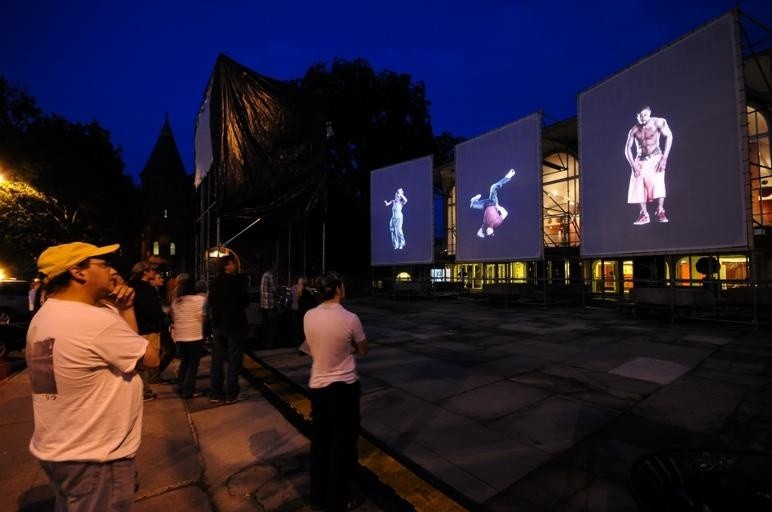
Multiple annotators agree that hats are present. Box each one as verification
[316,272,344,289]
[132,262,159,272]
[37,241,121,285]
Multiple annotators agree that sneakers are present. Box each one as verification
[633,211,651,225]
[654,207,669,223]
[141,389,241,405]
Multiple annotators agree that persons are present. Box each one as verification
[470,169,515,239]
[125,253,250,403]
[384,188,407,250]
[624,105,673,226]
[25,239,160,512]
[27,278,43,311]
[35,276,50,307]
[302,273,369,512]
[252,260,288,350]
[290,276,313,311]
